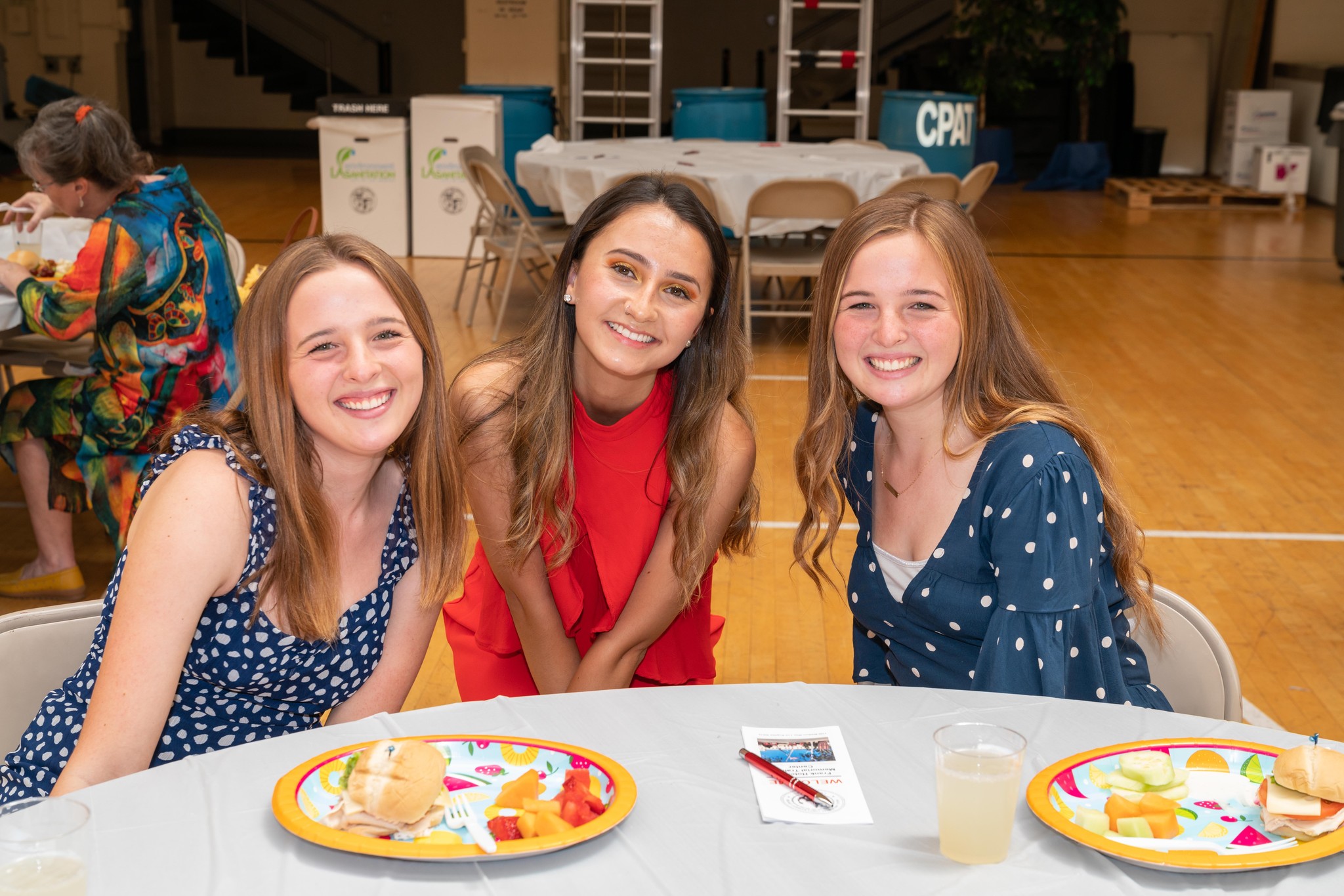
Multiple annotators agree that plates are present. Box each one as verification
[1027,737,1343,877]
[271,734,640,863]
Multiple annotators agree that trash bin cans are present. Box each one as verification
[671,88,768,236]
[462,84,554,222]
[880,91,980,213]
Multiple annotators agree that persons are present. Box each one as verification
[443,173,760,703]
[0,96,245,601]
[0,233,473,815]
[790,193,1174,718]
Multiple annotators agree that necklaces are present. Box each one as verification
[880,413,961,498]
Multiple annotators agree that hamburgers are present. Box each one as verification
[335,741,447,836]
[1258,744,1344,841]
[6,250,41,278]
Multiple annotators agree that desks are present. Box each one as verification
[516,137,932,329]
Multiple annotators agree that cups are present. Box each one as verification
[932,720,1027,864]
[0,796,92,895]
[11,219,43,275]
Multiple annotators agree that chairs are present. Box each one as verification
[449,131,1000,351]
[1126,581,1244,722]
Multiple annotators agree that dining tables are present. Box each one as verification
[0,681,1344,896]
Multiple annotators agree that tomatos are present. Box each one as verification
[487,768,606,841]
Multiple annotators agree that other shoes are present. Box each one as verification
[0,562,88,601]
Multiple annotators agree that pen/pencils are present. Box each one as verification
[738,749,835,810]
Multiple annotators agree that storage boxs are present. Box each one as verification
[1217,85,1312,195]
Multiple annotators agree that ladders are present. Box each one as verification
[569,0,664,138]
[776,0,873,143]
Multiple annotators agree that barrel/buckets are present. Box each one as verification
[878,88,978,211]
[461,84,557,218]
[671,86,767,142]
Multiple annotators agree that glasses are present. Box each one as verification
[32,180,57,192]
